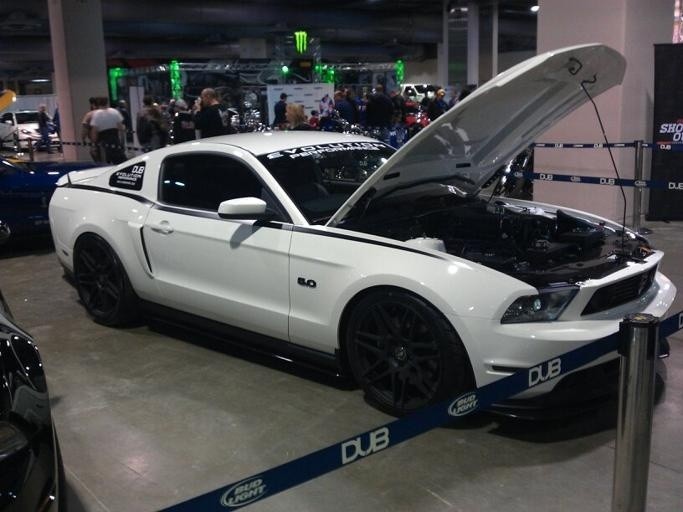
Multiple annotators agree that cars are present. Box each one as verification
[0,111,61,149]
[401,84,437,106]
[0,157,120,250]
[48,41,678,418]
[0,292,64,512]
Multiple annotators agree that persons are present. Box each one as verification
[81,86,233,158]
[274,83,477,150]
[34,103,64,154]
